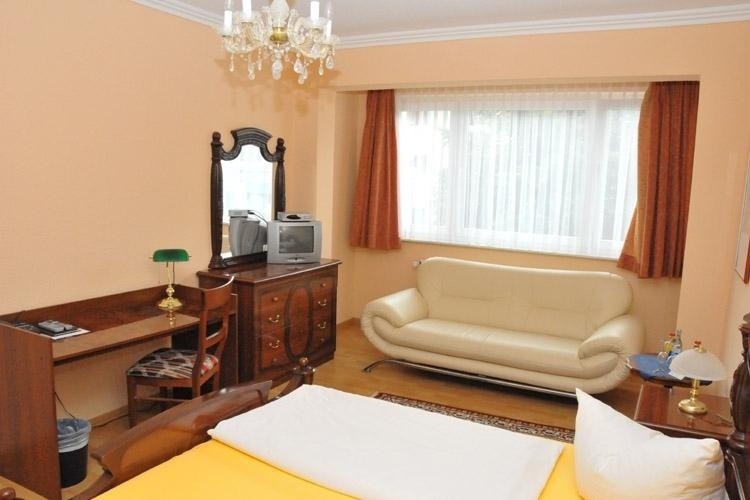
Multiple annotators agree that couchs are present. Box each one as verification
[359,256,646,399]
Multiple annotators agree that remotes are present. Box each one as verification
[38,321,64,333]
[48,319,73,330]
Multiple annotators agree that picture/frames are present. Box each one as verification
[734,159,750,284]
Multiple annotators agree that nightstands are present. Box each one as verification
[633,382,736,448]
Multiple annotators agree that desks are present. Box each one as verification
[0,283,239,500]
[624,352,713,389]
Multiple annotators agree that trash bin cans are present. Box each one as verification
[56,418,92,488]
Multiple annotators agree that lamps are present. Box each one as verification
[195,258,346,392]
[220,0,340,85]
[150,248,190,311]
[667,346,727,415]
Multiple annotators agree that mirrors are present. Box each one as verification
[208,127,287,270]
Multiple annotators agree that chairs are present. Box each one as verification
[122,272,236,430]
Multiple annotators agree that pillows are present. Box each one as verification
[573,386,731,500]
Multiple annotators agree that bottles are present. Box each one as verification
[663,328,682,356]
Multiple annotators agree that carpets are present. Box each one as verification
[370,390,576,445]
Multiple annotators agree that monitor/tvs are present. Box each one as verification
[229,214,267,257]
[267,219,322,264]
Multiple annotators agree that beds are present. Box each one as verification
[0,324,750,500]
